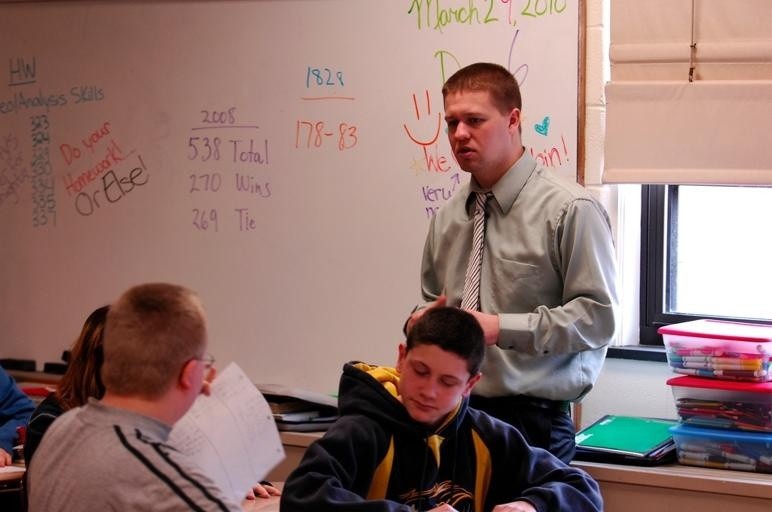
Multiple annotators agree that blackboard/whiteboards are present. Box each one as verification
[0,0,588,434]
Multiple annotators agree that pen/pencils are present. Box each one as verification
[677,437,772,474]
[675,398,772,433]
[664,336,772,383]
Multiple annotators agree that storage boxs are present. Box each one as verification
[658,316,772,473]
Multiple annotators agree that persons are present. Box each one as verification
[245,480,281,500]
[1,364,38,467]
[26,281,244,512]
[23,303,111,468]
[416,60,618,463]
[279,305,604,512]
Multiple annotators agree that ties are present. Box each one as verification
[460,193,495,312]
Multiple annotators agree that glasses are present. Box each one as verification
[192,352,214,368]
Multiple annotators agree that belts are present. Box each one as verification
[522,396,570,415]
